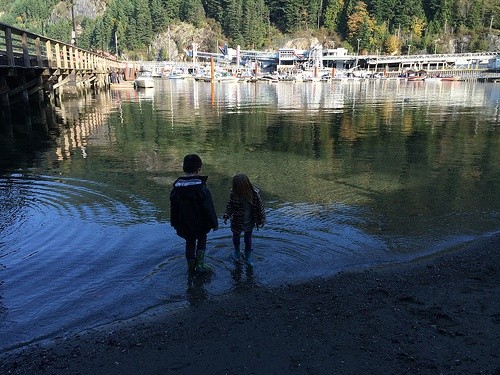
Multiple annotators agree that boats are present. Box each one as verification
[155,53,463,84]
[133,71,154,87]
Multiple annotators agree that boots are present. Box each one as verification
[195,250,210,272]
[187,259,194,271]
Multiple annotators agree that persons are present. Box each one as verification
[222,173,265,266]
[170,154,219,272]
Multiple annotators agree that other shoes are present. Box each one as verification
[235,250,252,258]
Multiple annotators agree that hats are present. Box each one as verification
[184,155,201,170]
